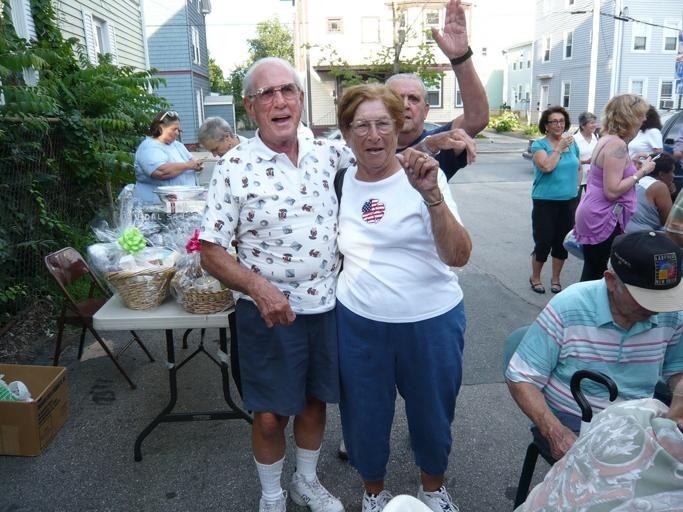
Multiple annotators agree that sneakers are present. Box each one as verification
[259,490,288,512]
[417,484,461,512]
[290,472,345,512]
[362,490,394,512]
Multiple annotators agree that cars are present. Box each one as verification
[661,114,681,185]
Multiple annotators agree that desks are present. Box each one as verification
[92,290,254,463]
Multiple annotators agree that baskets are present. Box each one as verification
[106,269,177,312]
[172,274,239,316]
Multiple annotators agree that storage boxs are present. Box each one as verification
[7,363,70,457]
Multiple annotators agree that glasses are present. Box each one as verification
[547,120,565,125]
[247,85,301,103]
[161,111,180,122]
[348,118,398,137]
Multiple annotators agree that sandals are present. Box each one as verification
[551,279,561,294]
[530,276,546,293]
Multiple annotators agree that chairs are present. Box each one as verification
[502,325,674,511]
[44,247,155,390]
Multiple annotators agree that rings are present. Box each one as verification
[422,153,430,162]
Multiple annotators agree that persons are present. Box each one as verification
[505,231,683,459]
[385,0,490,180]
[571,112,600,198]
[623,153,677,233]
[529,106,583,294]
[199,57,413,512]
[334,84,473,512]
[574,94,650,281]
[133,110,205,205]
[628,103,664,159]
[198,115,249,157]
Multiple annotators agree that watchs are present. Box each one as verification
[449,46,474,65]
[632,174,639,184]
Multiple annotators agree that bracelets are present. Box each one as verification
[553,148,563,156]
[423,193,444,208]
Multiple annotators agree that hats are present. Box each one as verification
[611,230,683,313]
